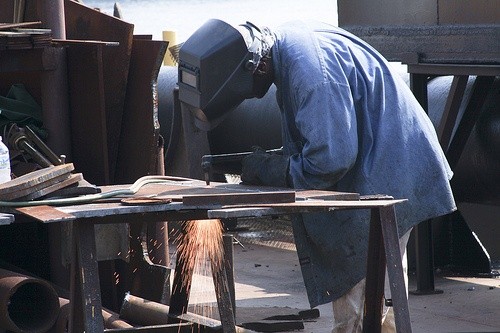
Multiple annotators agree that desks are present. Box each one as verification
[12,178,413,333]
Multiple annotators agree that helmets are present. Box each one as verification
[176,18,254,132]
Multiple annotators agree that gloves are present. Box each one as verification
[240,143,290,189]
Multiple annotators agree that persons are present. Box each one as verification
[174,14,459,333]
